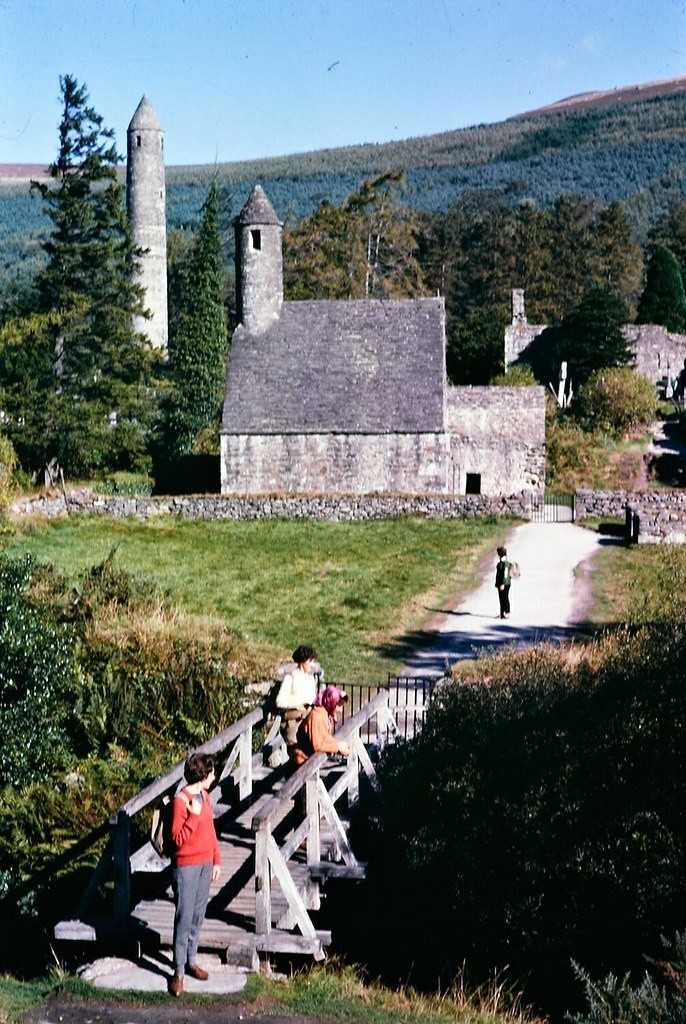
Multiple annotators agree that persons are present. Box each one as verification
[276,646,350,846]
[170,753,221,996]
[495,547,513,618]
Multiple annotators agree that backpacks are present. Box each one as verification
[151,788,206,859]
[503,561,521,578]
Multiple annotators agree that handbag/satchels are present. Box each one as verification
[262,716,290,768]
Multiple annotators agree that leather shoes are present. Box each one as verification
[185,966,208,980]
[170,974,183,996]
